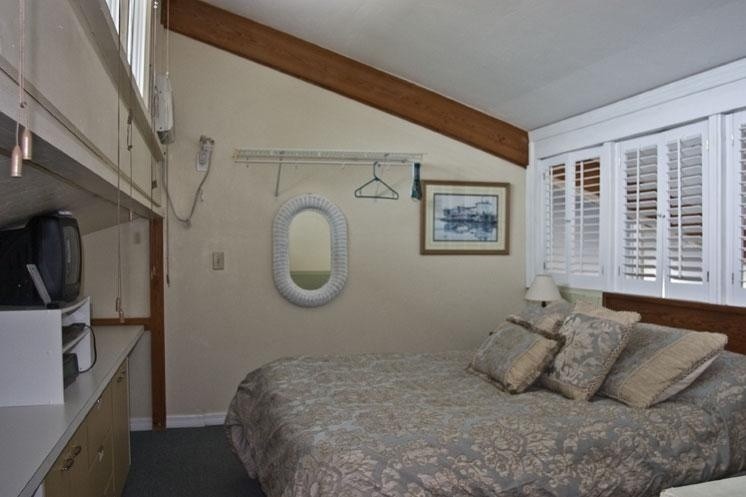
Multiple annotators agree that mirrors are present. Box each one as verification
[271,193,350,309]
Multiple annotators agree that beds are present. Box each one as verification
[224,292,746,497]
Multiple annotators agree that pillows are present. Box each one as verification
[465,300,729,412]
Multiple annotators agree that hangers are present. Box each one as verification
[354,159,399,200]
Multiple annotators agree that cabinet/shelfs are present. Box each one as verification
[44,358,132,497]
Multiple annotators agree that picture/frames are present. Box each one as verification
[419,179,511,256]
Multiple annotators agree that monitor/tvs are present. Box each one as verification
[0,214,83,309]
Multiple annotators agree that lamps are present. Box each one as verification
[525,274,564,310]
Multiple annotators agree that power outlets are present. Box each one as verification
[196,150,210,172]
